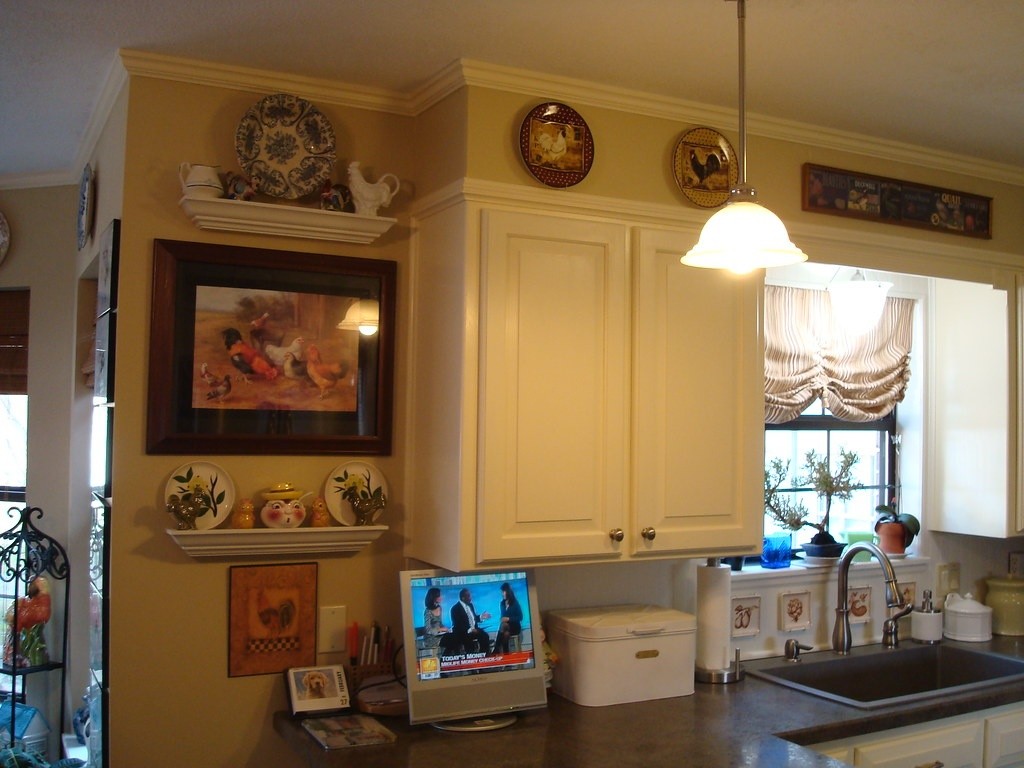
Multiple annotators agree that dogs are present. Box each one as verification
[301,671,330,699]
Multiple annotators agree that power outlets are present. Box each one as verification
[1007,551,1024,575]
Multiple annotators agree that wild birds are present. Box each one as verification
[4,575,53,660]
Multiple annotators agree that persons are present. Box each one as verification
[451,588,490,658]
[424,588,462,662]
[492,583,523,657]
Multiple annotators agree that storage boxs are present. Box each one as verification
[546,602,697,708]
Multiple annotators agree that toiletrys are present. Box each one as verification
[909,588,944,644]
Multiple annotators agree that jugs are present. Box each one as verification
[179,161,225,199]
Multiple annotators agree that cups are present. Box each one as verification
[760,532,792,569]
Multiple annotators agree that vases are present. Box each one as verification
[760,532,792,570]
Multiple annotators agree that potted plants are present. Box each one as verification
[874,497,921,554]
[763,444,864,557]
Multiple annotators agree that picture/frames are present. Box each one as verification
[96,218,122,319]
[92,311,116,406]
[804,161,995,241]
[145,238,398,458]
[226,560,318,679]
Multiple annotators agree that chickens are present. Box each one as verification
[163,483,207,531]
[688,149,722,186]
[536,126,569,170]
[342,485,387,527]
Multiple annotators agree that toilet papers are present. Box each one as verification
[696,563,732,671]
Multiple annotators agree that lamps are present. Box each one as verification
[679,0,808,277]
[827,267,895,336]
[336,298,380,336]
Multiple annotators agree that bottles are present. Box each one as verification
[984,573,1024,636]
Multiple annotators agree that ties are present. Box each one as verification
[466,605,474,628]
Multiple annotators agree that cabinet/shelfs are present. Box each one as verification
[926,273,1024,540]
[0,506,71,761]
[983,700,1024,768]
[851,711,985,768]
[400,177,768,574]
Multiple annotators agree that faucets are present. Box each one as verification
[832,540,904,656]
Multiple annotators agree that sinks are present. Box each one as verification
[757,641,1024,711]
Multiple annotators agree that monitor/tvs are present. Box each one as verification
[400,570,548,731]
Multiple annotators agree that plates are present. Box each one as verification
[235,91,335,200]
[165,460,235,530]
[673,126,739,206]
[326,461,386,524]
[520,101,595,190]
